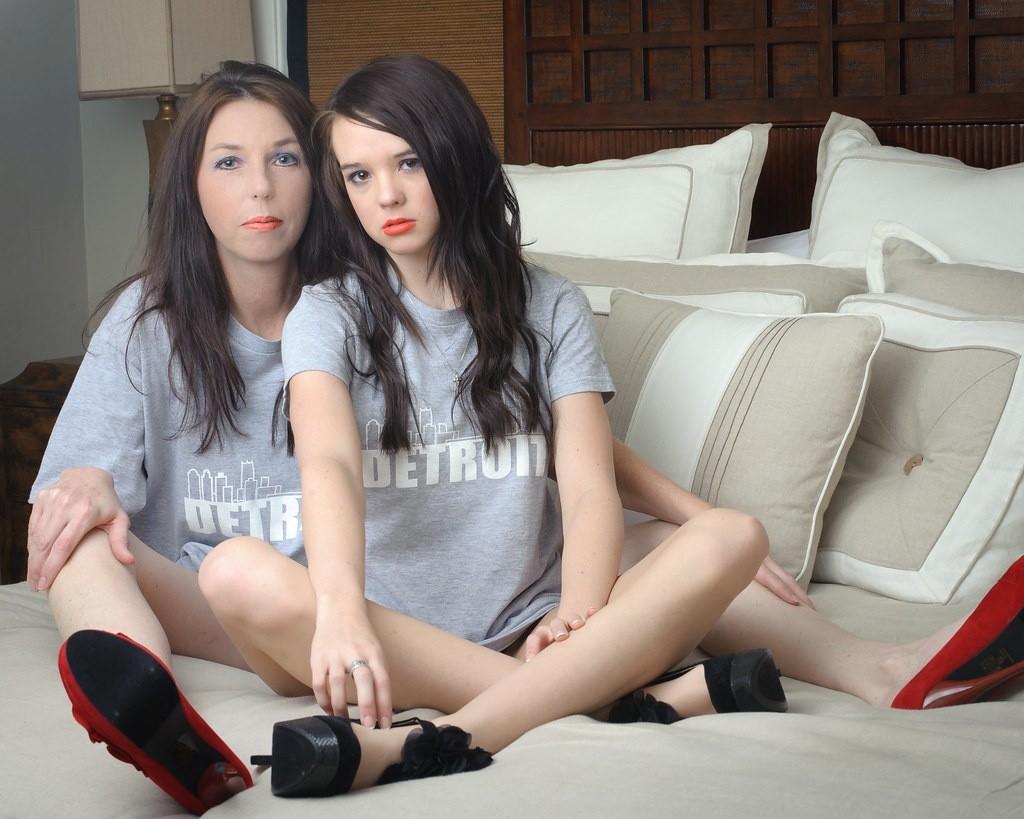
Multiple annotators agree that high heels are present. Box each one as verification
[892,553,1024,710]
[250,715,491,799]
[59,629,254,816]
[586,648,787,724]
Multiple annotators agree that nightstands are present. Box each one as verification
[0,355,86,586]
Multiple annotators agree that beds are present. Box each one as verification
[0,106,1024,817]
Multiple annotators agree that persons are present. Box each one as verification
[27,62,1024,816]
[199,53,788,797]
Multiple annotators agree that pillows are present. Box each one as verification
[589,289,888,600]
[499,122,774,256]
[875,221,1024,314]
[788,293,1024,602]
[804,107,1024,275]
[522,254,871,315]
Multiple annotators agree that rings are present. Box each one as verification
[350,660,368,679]
